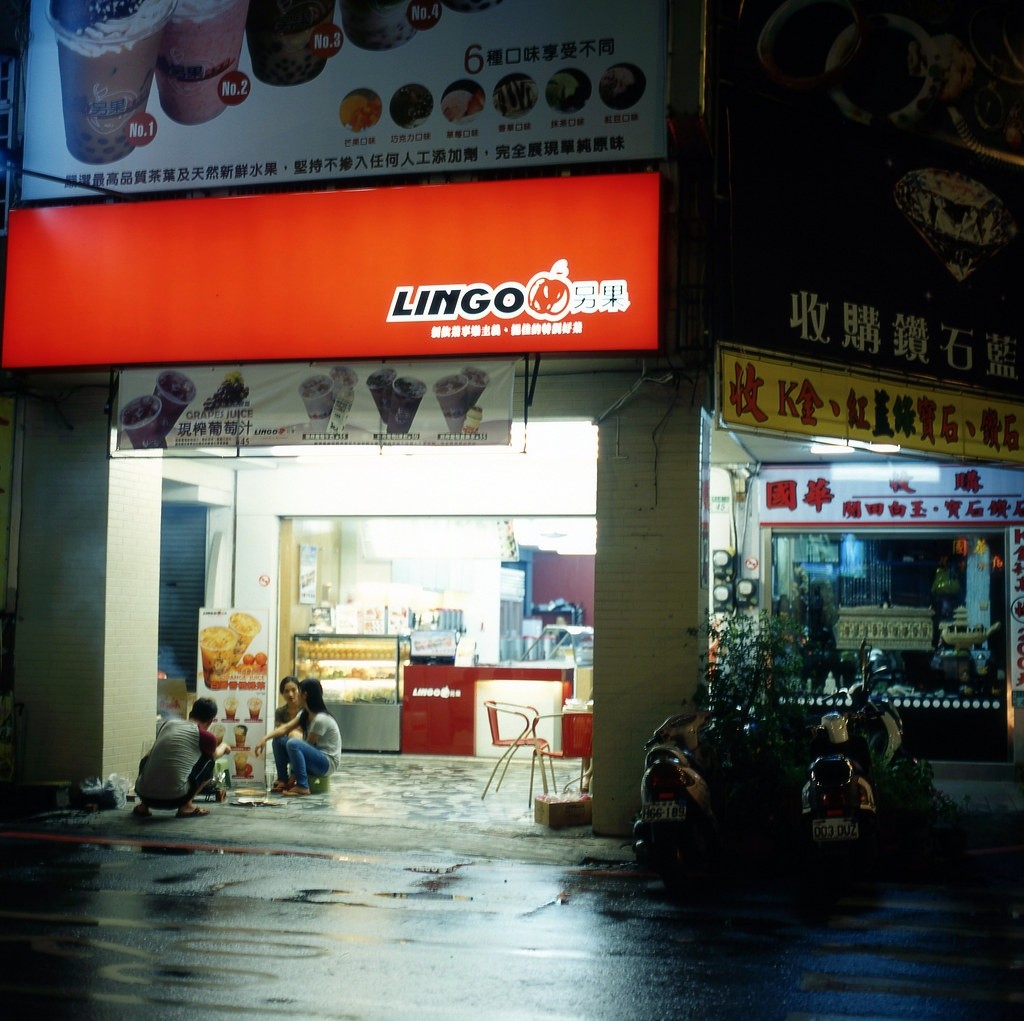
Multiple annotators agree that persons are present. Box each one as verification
[254,678,342,796]
[133,697,233,817]
[274,676,301,729]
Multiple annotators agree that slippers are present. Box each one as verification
[176,806,209,818]
[133,805,152,816]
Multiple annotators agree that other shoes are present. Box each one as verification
[284,787,310,796]
[272,781,287,792]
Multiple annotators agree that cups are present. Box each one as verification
[201,612,264,779]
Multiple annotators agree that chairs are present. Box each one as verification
[532,712,593,796]
[481,700,557,810]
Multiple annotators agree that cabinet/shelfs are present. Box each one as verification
[292,631,411,752]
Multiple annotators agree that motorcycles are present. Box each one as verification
[800,710,886,893]
[634,708,731,881]
[822,639,918,777]
